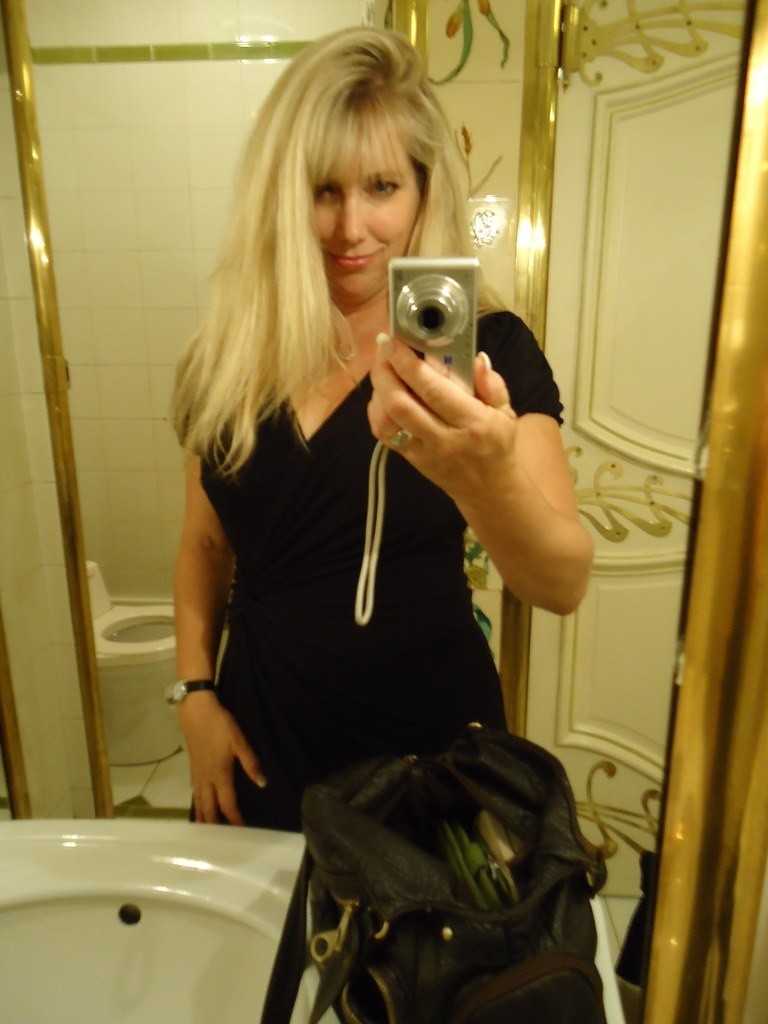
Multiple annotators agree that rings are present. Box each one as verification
[389,429,413,450]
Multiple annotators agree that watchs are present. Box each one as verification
[163,677,216,703]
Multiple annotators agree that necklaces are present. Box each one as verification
[337,340,356,360]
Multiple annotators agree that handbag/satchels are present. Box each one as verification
[261,725,615,1023]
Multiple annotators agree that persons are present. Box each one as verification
[161,25,593,922]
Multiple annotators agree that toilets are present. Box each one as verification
[84,559,183,765]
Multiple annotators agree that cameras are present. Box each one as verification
[390,256,480,397]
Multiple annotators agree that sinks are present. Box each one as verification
[0,819,340,1024]
